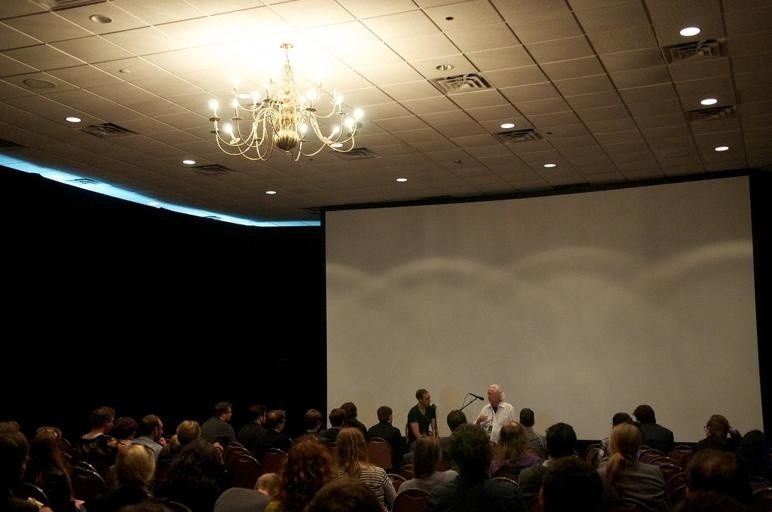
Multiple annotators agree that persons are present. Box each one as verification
[473,384,519,444]
[0,402,772,511]
[404,388,439,439]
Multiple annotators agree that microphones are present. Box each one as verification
[469,392,484,401]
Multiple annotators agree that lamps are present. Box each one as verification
[206,37,367,163]
